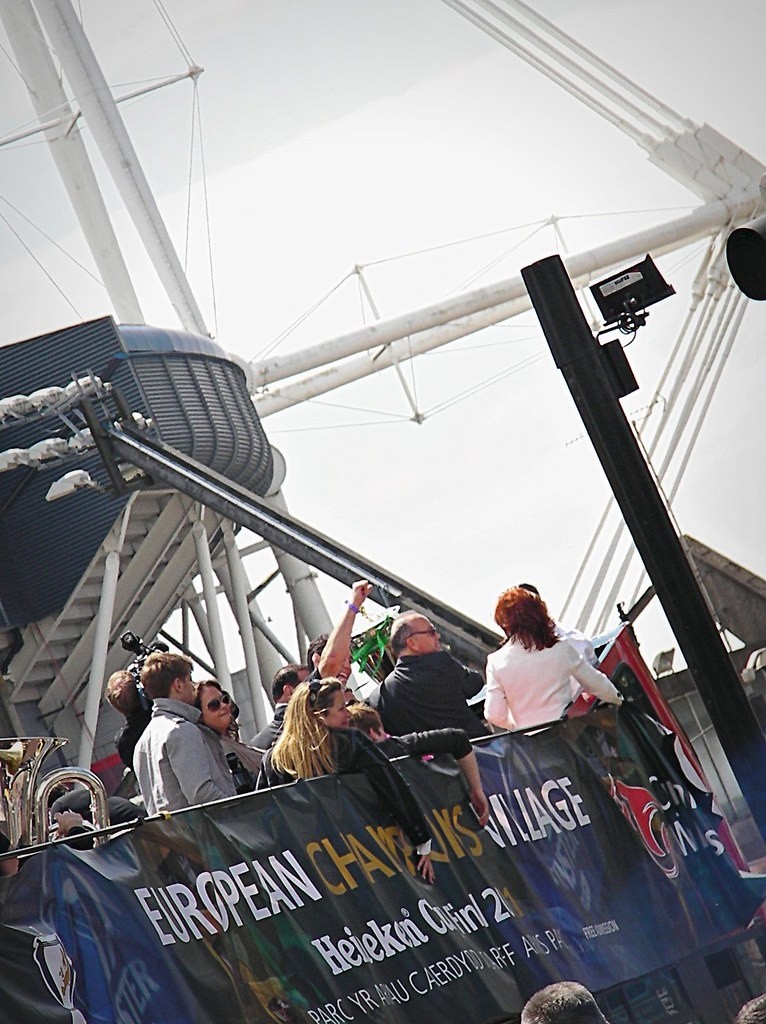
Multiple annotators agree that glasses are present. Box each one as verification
[406,627,437,640]
[206,695,230,712]
[187,675,192,681]
[344,700,361,707]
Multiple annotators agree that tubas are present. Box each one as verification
[0,736,111,852]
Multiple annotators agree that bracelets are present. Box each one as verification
[345,600,359,614]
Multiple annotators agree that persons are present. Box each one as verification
[255,676,435,884]
[368,609,493,741]
[132,651,238,817]
[520,981,609,1024]
[0,579,766,1024]
[483,586,625,732]
